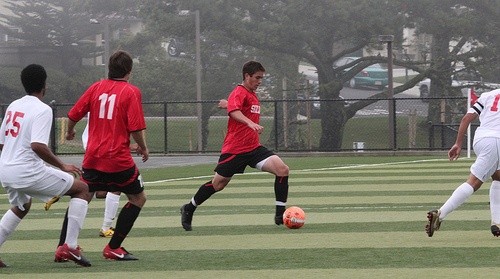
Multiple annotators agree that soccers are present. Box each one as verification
[282,206,305,229]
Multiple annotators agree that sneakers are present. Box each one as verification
[274,216,283,224]
[180,203,193,230]
[99,226,115,237]
[425,209,438,236]
[103,243,139,260]
[61,242,92,266]
[0,259,7,266]
[55,245,69,262]
[490,225,500,236]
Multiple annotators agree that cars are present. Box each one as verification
[418,66,500,115]
[308,79,349,109]
[332,56,389,89]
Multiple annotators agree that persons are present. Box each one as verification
[54,51,150,262]
[179,61,290,231]
[0,64,92,268]
[425,89,500,238]
